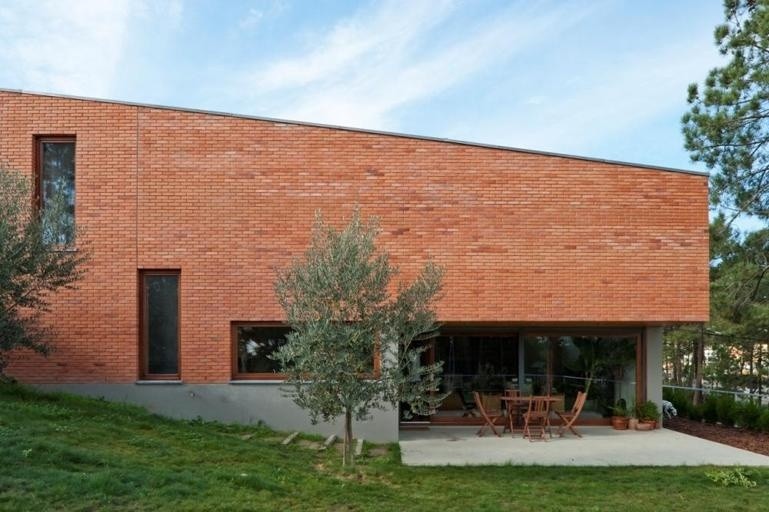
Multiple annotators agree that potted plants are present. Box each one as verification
[606,394,660,431]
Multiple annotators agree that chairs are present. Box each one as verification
[453,388,478,418]
[471,389,587,442]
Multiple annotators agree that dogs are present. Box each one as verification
[663,399,677,419]
